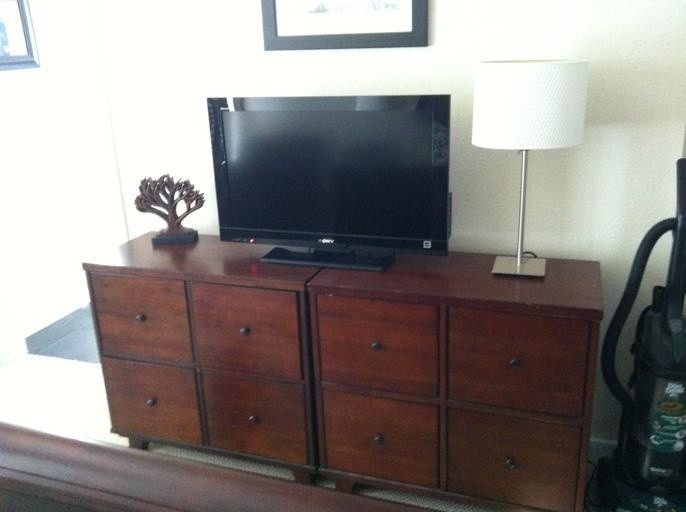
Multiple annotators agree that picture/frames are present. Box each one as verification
[0,0,40,71]
[261,0,428,50]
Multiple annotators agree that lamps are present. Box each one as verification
[470,59,588,279]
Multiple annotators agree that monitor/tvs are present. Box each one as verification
[203,93,452,274]
